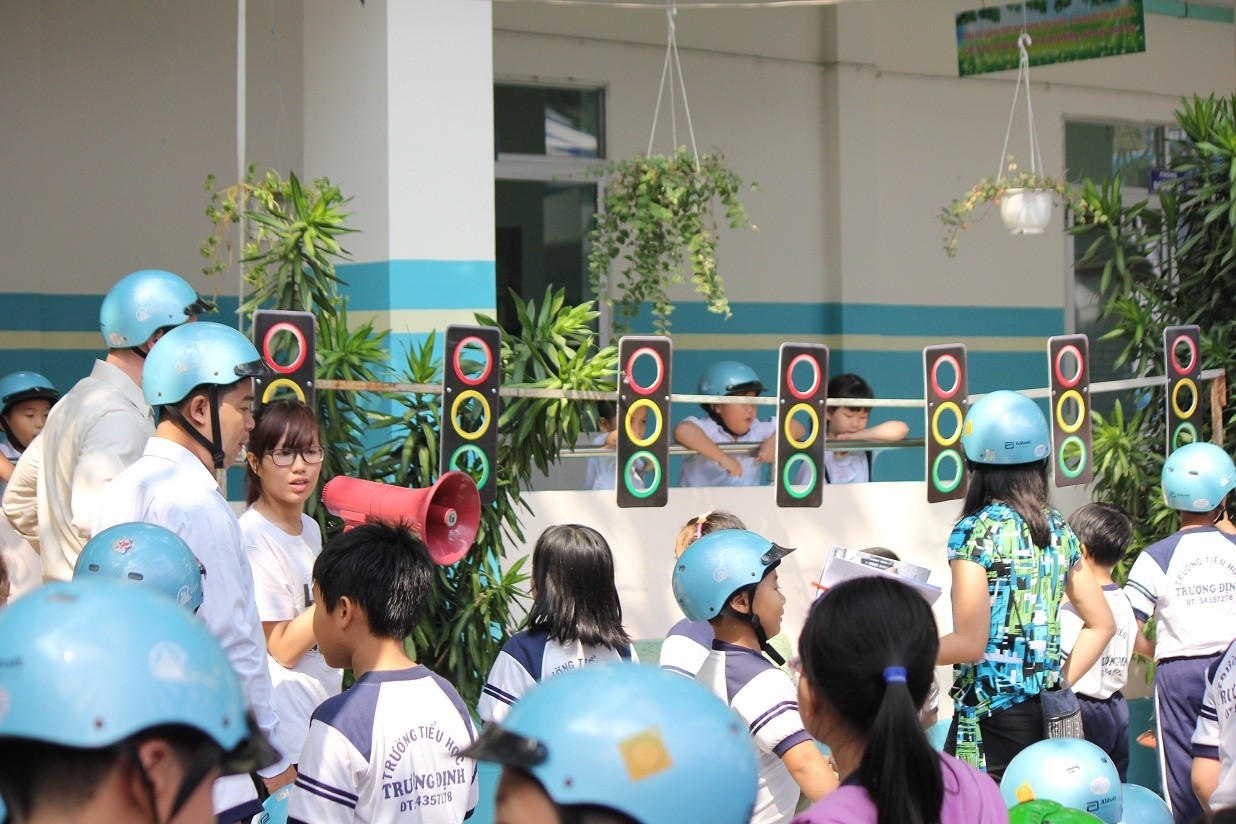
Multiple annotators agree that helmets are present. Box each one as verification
[698,361,769,396]
[449,659,759,824]
[1001,737,1176,824]
[0,562,251,752]
[141,321,272,405]
[0,371,61,417]
[72,522,207,616]
[1161,441,1236,512]
[960,390,1051,464]
[99,271,216,348]
[672,529,799,622]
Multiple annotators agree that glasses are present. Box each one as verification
[260,446,326,468]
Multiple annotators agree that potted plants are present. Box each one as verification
[582,148,763,344]
[938,157,1088,264]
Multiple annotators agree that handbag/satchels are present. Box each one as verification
[1037,688,1086,740]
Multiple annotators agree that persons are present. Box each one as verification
[1120,441,1236,823]
[1,267,215,586]
[584,387,648,489]
[822,373,909,486]
[931,389,1116,786]
[0,513,1235,824]
[672,360,777,486]
[232,399,344,782]
[1057,502,1138,783]
[0,369,59,507]
[92,320,296,823]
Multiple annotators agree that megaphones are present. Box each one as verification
[322,471,481,566]
